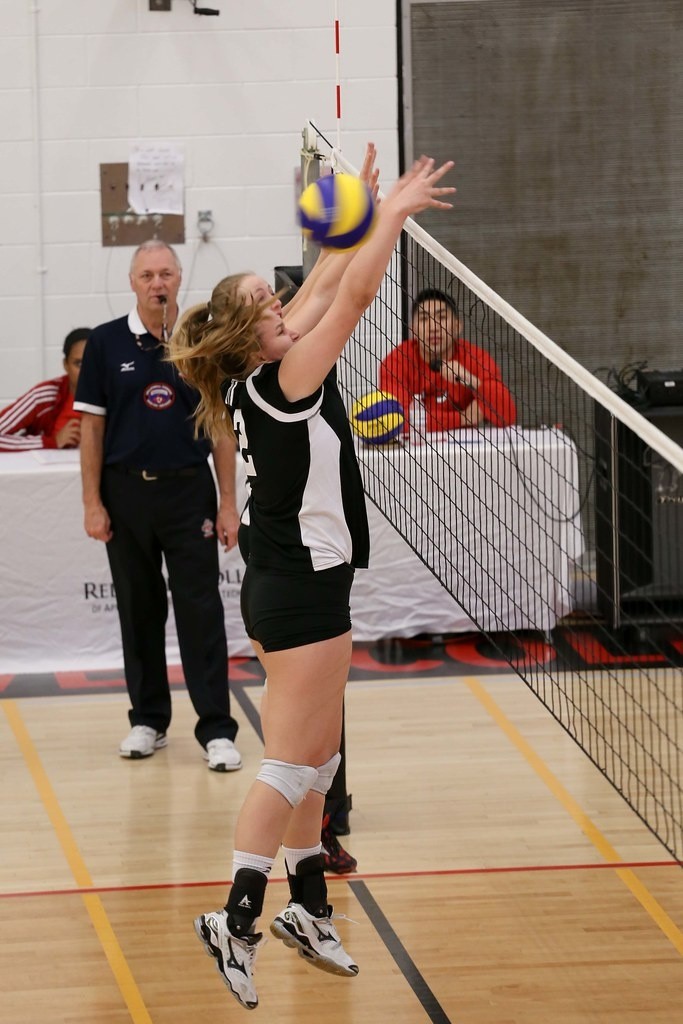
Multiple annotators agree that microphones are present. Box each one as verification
[429,360,472,388]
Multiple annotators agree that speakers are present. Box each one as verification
[595,405,683,617]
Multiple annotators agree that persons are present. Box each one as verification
[73,243,241,771]
[379,291,515,429]
[192,158,459,1008]
[0,328,90,452]
[172,143,383,876]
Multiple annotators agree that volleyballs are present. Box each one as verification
[349,390,405,444]
[296,173,379,255]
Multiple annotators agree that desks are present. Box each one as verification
[1,426,588,674]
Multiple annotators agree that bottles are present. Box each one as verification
[410,394,425,446]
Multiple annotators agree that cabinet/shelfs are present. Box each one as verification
[595,402,681,645]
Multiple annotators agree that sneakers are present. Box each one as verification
[193,908,269,1010]
[270,900,359,977]
[319,811,358,874]
[202,737,243,772]
[119,723,168,758]
